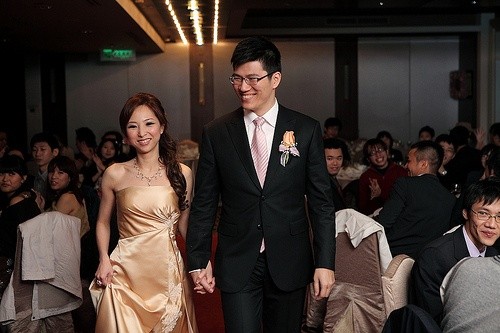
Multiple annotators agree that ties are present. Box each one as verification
[251,117,269,188]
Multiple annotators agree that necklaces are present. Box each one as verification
[133,159,164,186]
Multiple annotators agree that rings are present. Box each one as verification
[97,280,102,286]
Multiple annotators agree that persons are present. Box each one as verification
[88,93,212,333]
[186,38,336,333]
[0,128,126,298]
[323,117,500,333]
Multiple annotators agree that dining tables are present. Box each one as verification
[337,163,367,188]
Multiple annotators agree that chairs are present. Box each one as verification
[0,208,460,333]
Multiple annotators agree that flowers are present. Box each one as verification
[276,131,300,168]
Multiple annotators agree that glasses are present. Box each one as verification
[470,208,500,223]
[229,71,273,85]
[367,147,385,157]
